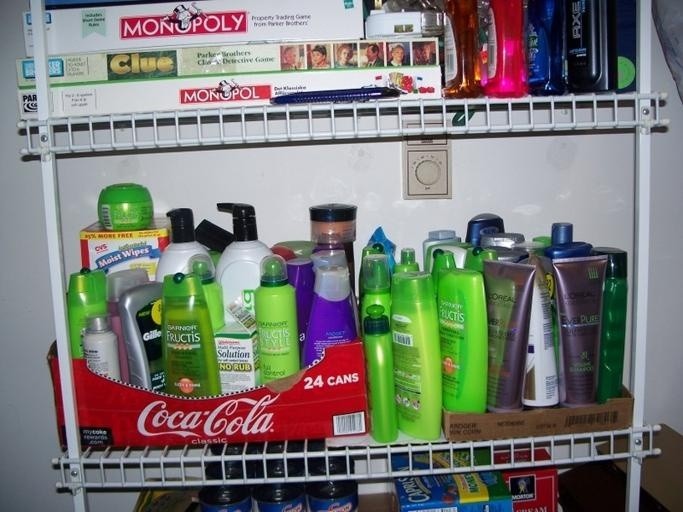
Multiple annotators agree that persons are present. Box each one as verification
[389,43,405,66]
[362,44,383,67]
[282,46,304,69]
[412,40,435,65]
[311,45,330,67]
[335,44,355,66]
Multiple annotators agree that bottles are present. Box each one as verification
[199,440,360,512]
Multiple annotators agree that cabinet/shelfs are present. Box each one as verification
[18,0,669,512]
